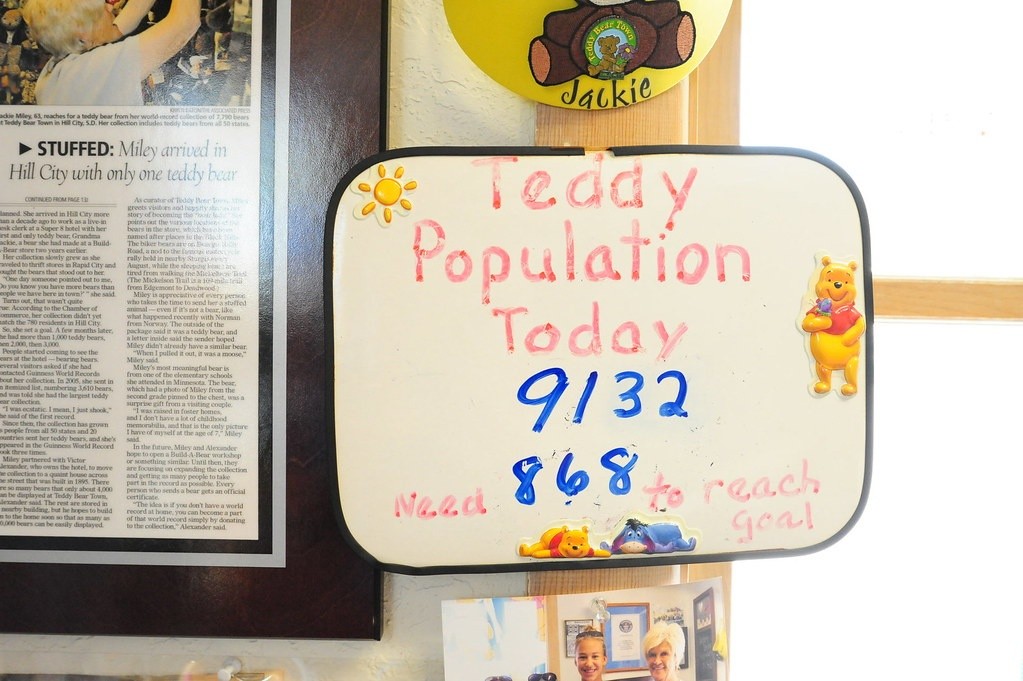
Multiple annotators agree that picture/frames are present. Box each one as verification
[600,603,649,673]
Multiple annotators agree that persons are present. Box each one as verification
[574,625,609,681]
[22,0,201,106]
[642,620,686,681]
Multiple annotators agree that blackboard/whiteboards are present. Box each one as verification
[323,145,876,576]
